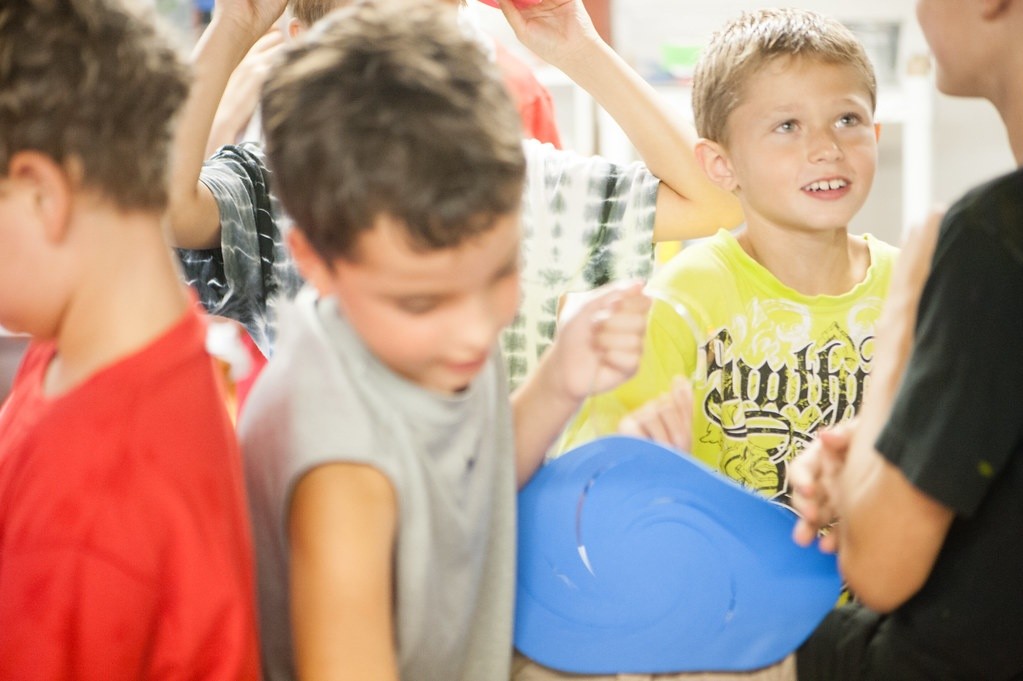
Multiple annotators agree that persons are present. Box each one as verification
[559,5,905,609]
[0,1,268,681]
[165,1,749,403]
[198,2,610,180]
[784,0,1023,680]
[235,4,651,681]
